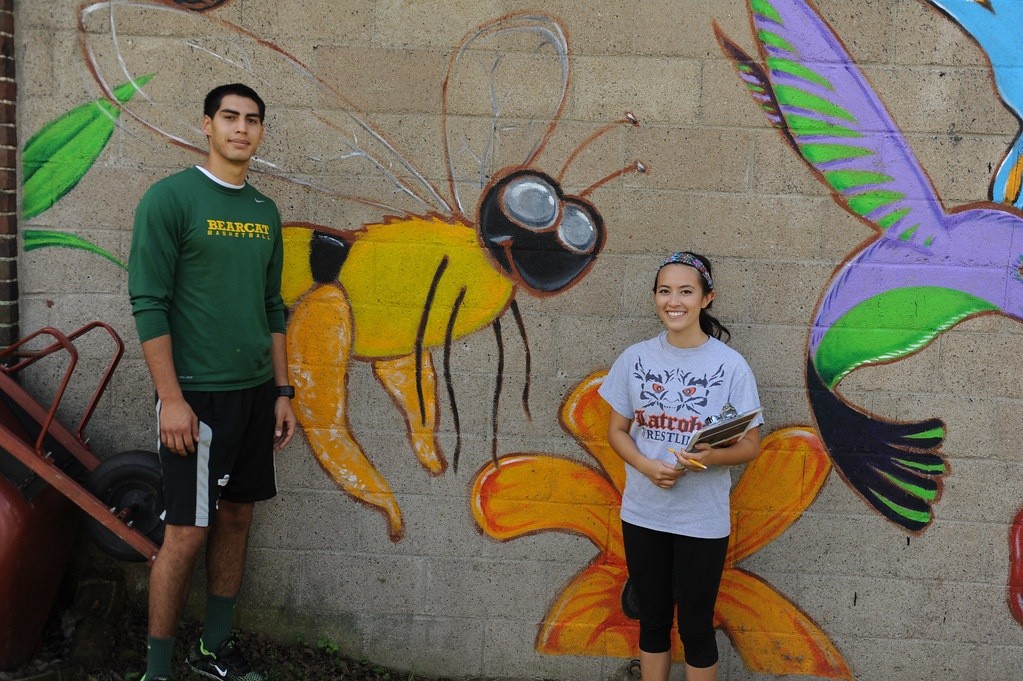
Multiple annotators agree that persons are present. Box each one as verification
[127,83,296,680]
[596,251,764,681]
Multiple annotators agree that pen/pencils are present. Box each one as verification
[664,447,708,470]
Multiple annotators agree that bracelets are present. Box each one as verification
[277,386,295,399]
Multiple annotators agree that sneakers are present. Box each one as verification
[140,673,174,681]
[184,635,263,681]
